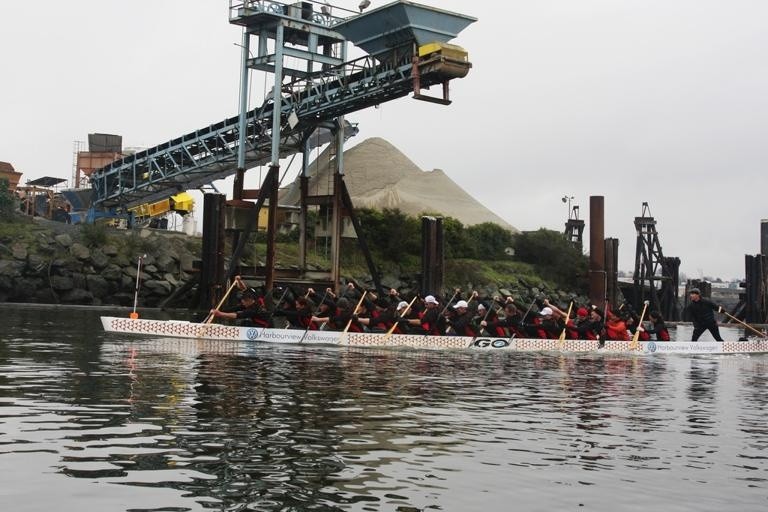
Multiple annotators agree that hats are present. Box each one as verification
[396,294,486,316]
[541,307,553,316]
[688,288,701,294]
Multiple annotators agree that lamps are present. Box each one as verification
[356,0,371,13]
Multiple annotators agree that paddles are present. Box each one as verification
[473,296,537,349]
[558,300,648,352]
[247,288,365,343]
[382,291,471,355]
[203,280,236,327]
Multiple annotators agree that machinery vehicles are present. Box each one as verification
[66,193,194,231]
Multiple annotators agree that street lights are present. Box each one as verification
[562,194,575,219]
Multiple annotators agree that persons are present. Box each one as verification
[210,276,275,328]
[264,285,671,341]
[686,288,726,341]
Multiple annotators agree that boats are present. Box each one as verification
[99,254,768,354]
